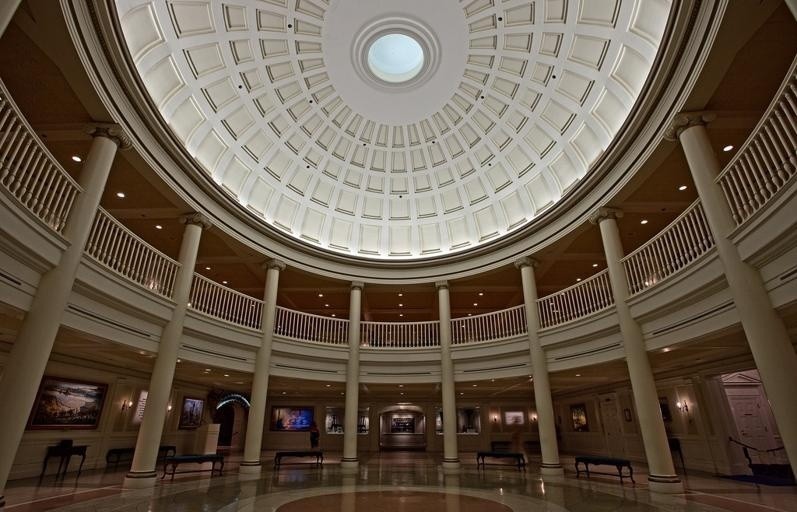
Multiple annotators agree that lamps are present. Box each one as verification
[121,399,133,411]
[677,400,688,415]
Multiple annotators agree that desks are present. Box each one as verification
[38,445,90,482]
[669,439,686,472]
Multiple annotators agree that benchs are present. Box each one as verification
[106,446,176,471]
[477,451,526,472]
[273,451,323,470]
[161,455,224,482]
[575,456,636,485]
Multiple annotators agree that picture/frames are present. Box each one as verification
[25,375,108,431]
[178,396,205,430]
[268,405,317,432]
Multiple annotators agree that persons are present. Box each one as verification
[310,422,321,448]
